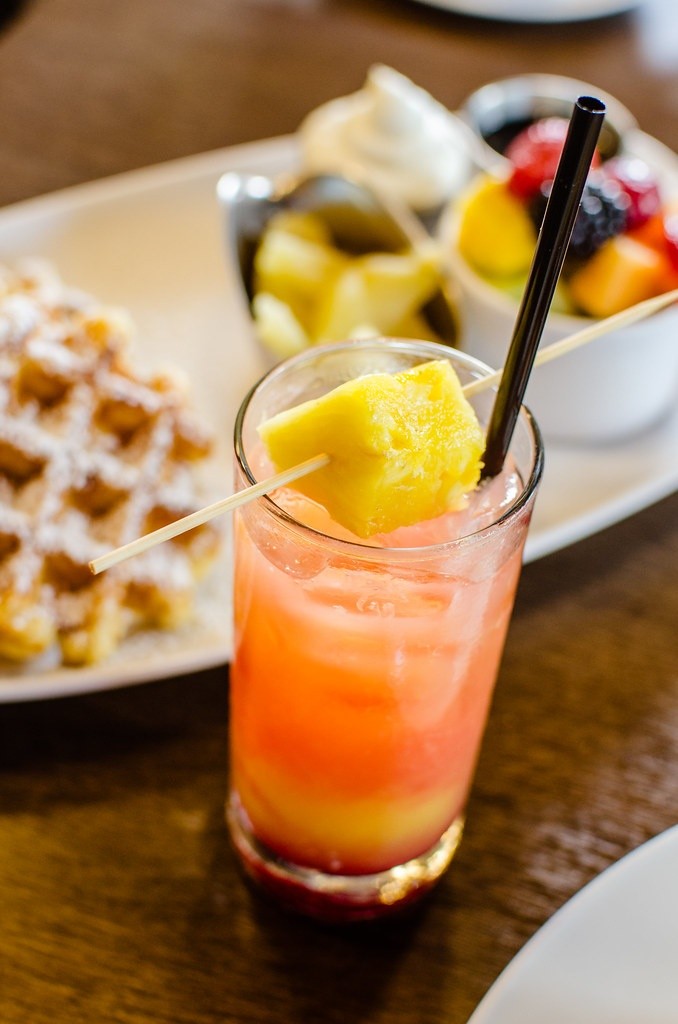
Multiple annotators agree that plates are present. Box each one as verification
[0,105,678,701]
[466,821,678,1024]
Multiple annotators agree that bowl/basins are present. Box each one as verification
[458,74,640,193]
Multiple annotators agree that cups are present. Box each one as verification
[218,337,546,915]
[441,160,678,448]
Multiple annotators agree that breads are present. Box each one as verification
[1,252,231,670]
